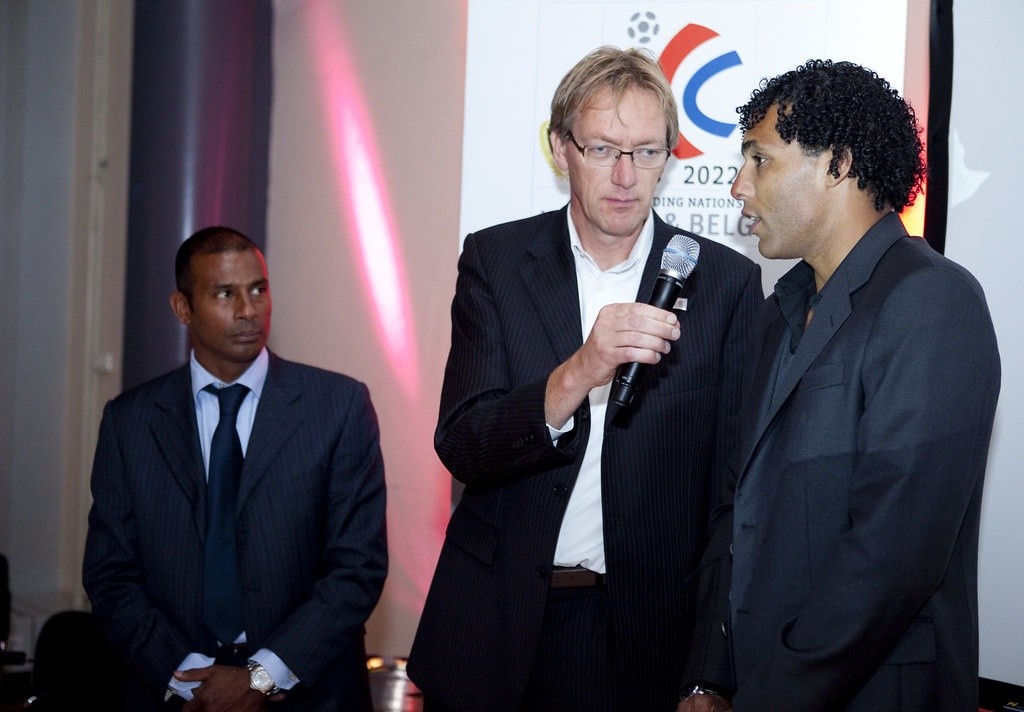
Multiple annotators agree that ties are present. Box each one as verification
[202,384,251,647]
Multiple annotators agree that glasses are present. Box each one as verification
[566,129,672,169]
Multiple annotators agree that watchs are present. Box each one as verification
[678,682,720,702]
[246,659,281,695]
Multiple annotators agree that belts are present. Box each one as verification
[549,568,606,589]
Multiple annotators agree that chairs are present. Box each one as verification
[0,610,99,712]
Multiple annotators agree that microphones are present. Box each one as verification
[610,234,700,408]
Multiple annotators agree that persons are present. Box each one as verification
[81,225,393,712]
[403,41,763,711]
[714,58,1004,710]
